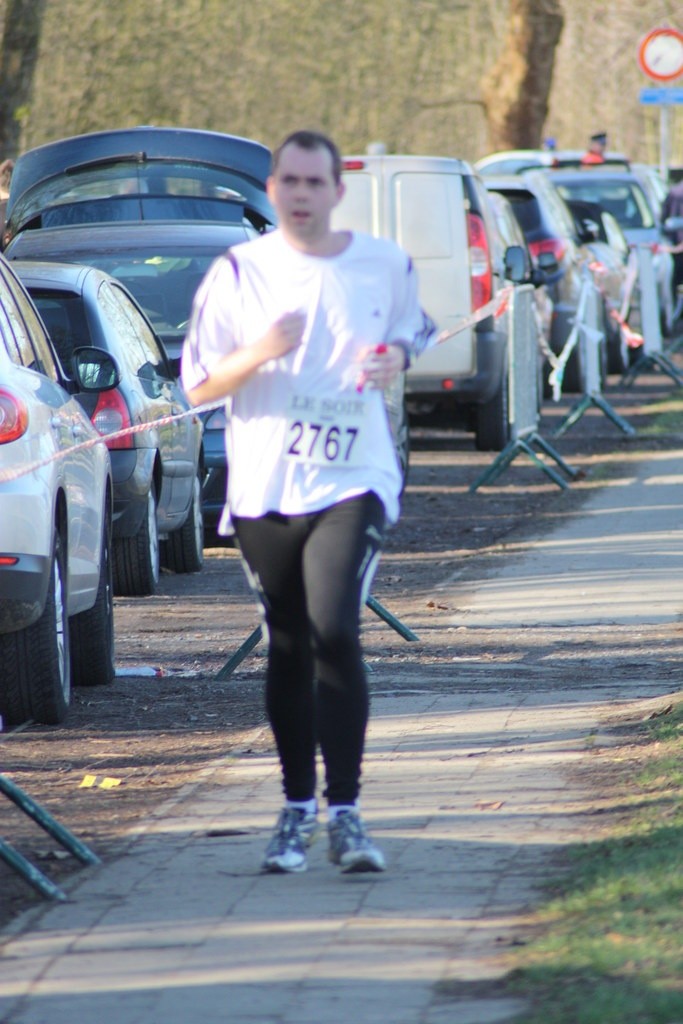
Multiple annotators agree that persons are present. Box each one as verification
[660,168,683,293]
[582,133,607,163]
[0,158,41,255]
[181,129,438,874]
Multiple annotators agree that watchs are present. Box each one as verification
[392,339,417,371]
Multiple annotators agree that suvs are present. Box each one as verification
[1,126,683,552]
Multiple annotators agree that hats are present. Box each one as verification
[590,133,607,145]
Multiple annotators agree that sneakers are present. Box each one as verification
[325,810,387,874]
[261,808,319,874]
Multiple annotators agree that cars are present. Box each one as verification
[0,250,125,724]
[10,256,204,599]
